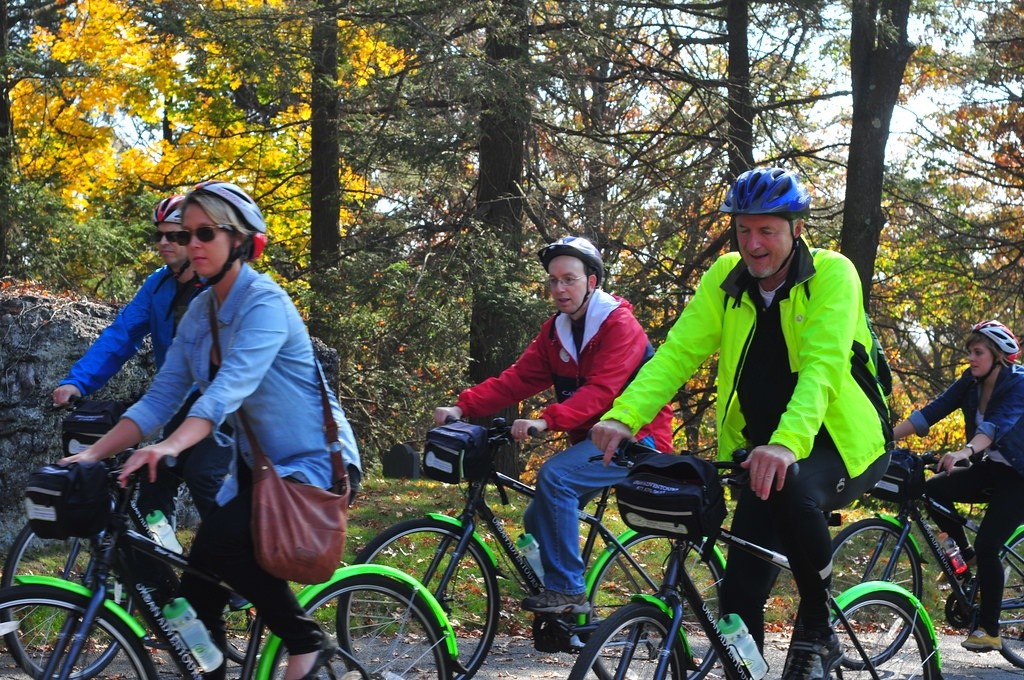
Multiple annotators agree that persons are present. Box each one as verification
[892,320,1024,649]
[591,169,896,680]
[435,237,674,613]
[53,182,362,680]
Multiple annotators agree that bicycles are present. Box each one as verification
[829,449,1023,670]
[0,395,468,680]
[337,414,726,680]
[568,429,941,680]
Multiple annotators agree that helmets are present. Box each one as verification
[538,237,602,288]
[973,320,1022,365]
[719,168,811,214]
[195,181,266,261]
[154,194,186,226]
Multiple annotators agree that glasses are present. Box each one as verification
[175,226,235,247]
[153,231,177,242]
[545,274,589,287]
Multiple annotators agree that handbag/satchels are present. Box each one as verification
[250,457,350,585]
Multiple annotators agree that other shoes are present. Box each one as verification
[297,630,336,680]
[230,594,254,610]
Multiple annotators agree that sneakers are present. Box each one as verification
[521,587,590,613]
[961,624,1002,650]
[935,554,976,585]
[781,624,844,680]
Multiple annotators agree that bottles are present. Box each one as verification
[938,533,968,574]
[146,506,183,556]
[162,597,223,672]
[716,614,768,680]
[516,533,546,587]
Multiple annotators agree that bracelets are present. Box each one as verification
[966,444,975,455]
